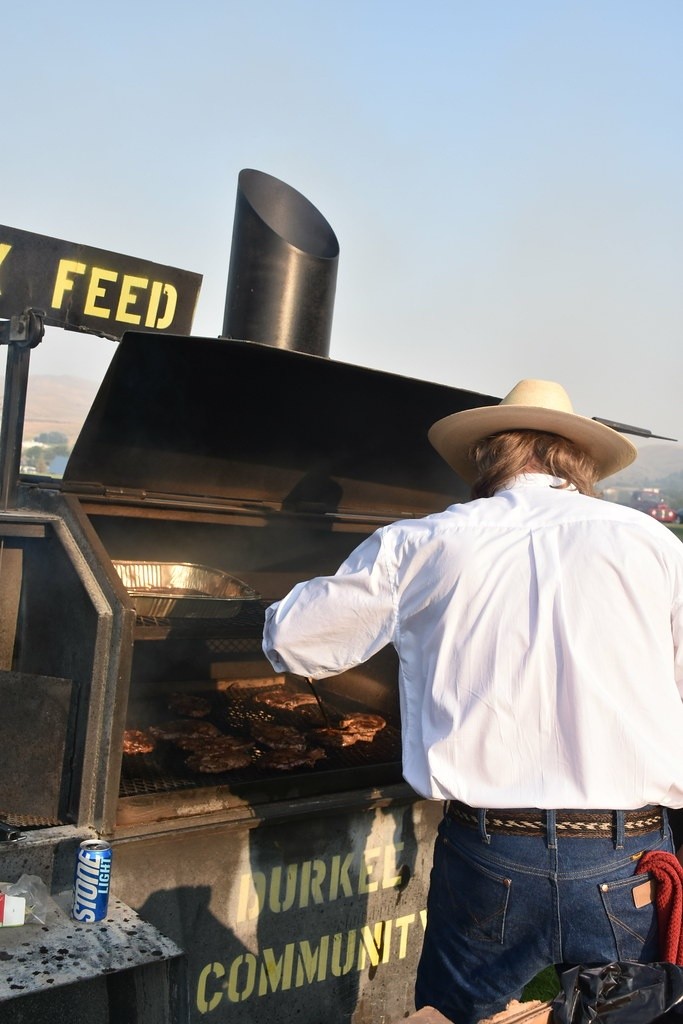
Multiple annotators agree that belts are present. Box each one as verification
[449,800,662,839]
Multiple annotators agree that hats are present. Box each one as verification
[428,379,638,485]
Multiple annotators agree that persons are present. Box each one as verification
[263,375,683,1024]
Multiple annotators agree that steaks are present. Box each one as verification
[121,689,386,775]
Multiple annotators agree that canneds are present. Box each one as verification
[70,839,113,924]
